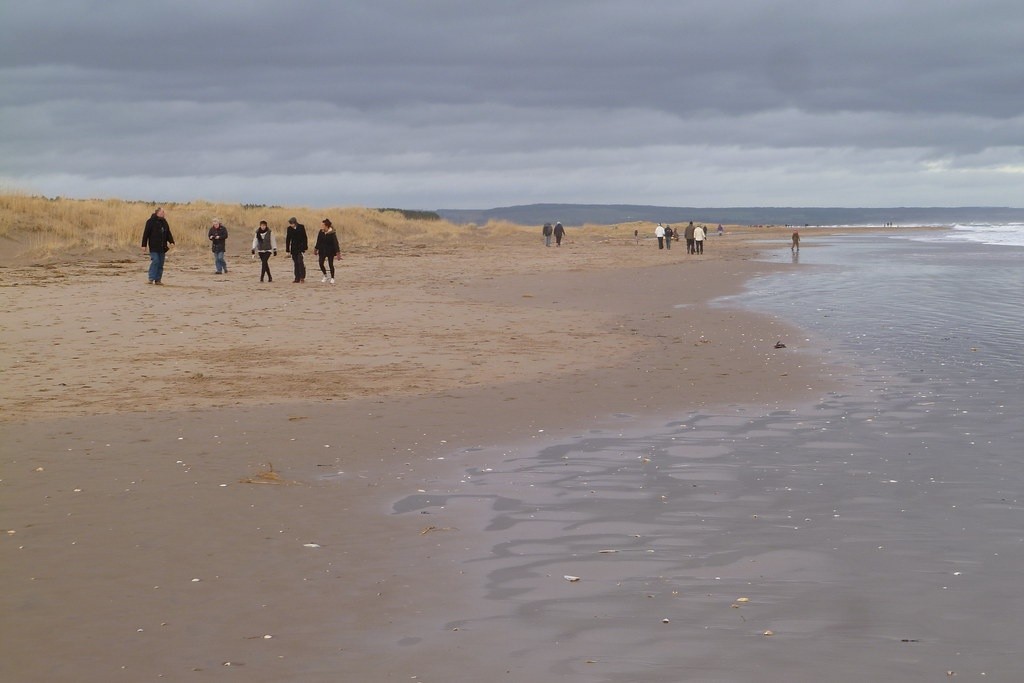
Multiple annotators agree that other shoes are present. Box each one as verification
[322,276,327,282]
[155,281,163,285]
[148,281,153,284]
[260,278,263,281]
[329,278,334,284]
[216,271,222,274]
[300,278,304,283]
[268,277,272,282]
[293,280,300,283]
[225,269,228,273]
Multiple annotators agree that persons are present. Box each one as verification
[252,221,278,282]
[747,223,809,251]
[717,224,723,236]
[208,219,228,275]
[684,221,695,254]
[554,221,565,247]
[285,216,308,284]
[703,225,707,235]
[883,221,892,227]
[543,222,553,247]
[314,219,341,283]
[141,207,174,285]
[693,223,706,255]
[664,224,672,250]
[655,222,665,249]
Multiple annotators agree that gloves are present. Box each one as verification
[337,252,341,260]
[252,249,255,254]
[313,248,318,255]
[273,249,277,256]
